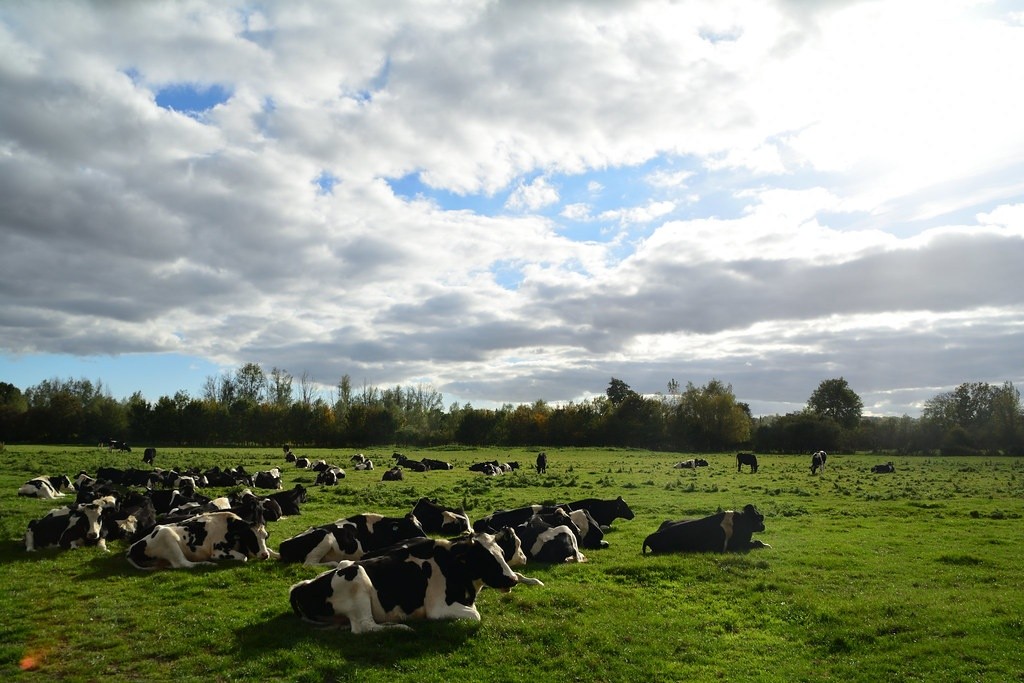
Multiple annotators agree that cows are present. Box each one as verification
[735,453,760,473]
[289,533,521,635]
[809,451,827,474]
[674,458,708,469]
[643,503,773,552]
[873,463,894,474]
[126,511,281,570]
[19,440,634,587]
